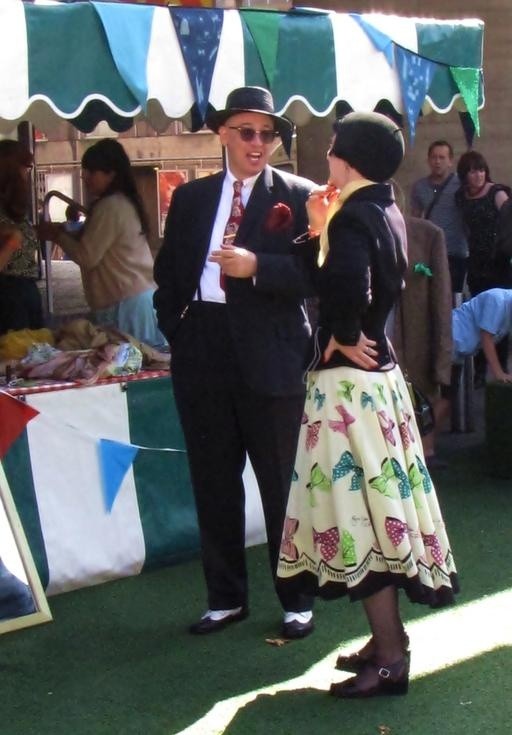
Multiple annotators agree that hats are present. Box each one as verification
[204,86,292,136]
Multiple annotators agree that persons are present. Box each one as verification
[409,140,473,310]
[382,177,453,414]
[33,138,172,355]
[159,173,184,236]
[51,204,84,260]
[273,110,462,700]
[492,197,511,376]
[1,140,34,175]
[453,149,511,390]
[419,286,512,470]
[0,140,46,334]
[150,83,318,640]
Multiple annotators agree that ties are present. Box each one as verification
[220,181,244,291]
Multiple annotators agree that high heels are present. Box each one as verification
[330,660,407,697]
[337,648,409,671]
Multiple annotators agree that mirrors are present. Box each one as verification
[0,463,56,634]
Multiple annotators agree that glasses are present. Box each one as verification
[228,126,280,144]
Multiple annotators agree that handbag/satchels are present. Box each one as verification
[407,379,434,435]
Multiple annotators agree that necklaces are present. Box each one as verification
[467,181,486,196]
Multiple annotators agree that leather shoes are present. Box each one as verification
[283,610,314,638]
[190,605,248,634]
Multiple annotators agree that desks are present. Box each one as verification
[0,355,268,597]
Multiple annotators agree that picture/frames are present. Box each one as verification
[156,169,190,239]
[180,120,213,134]
[194,166,220,180]
